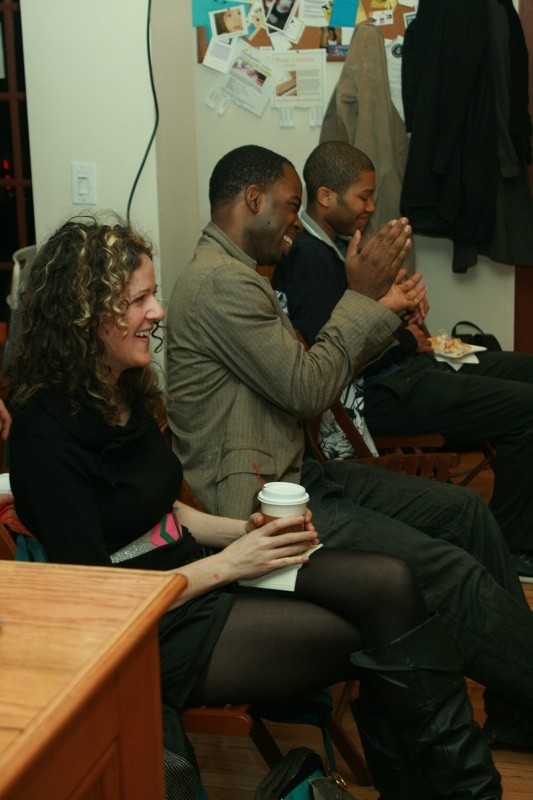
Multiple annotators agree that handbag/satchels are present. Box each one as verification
[449,321,502,352]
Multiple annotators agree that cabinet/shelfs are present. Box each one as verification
[0,556,188,800]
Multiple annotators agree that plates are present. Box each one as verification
[434,344,487,359]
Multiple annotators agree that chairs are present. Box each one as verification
[182,326,498,780]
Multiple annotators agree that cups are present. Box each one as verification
[258,481,310,538]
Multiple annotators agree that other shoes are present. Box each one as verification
[478,718,533,755]
[510,551,533,584]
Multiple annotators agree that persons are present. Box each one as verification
[0,399,12,440]
[164,144,533,750]
[3,210,503,800]
[272,140,533,582]
